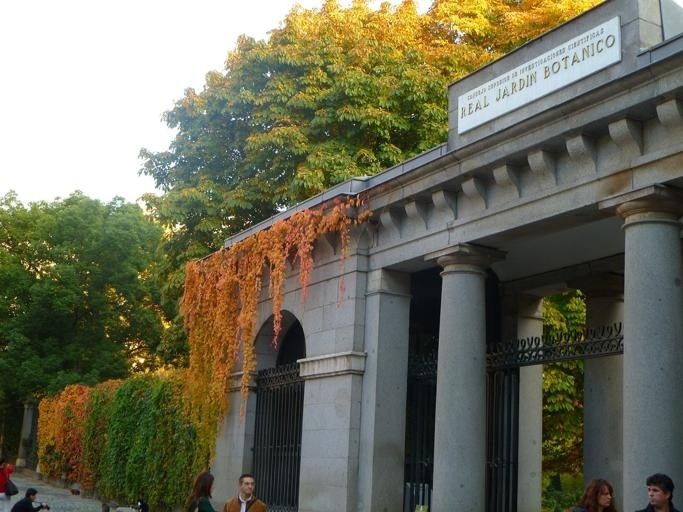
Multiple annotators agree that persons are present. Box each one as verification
[11,488,48,512]
[634,473,682,512]
[188,472,216,512]
[224,474,266,512]
[0,456,14,512]
[571,479,619,512]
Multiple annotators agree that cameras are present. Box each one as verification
[41,503,50,510]
